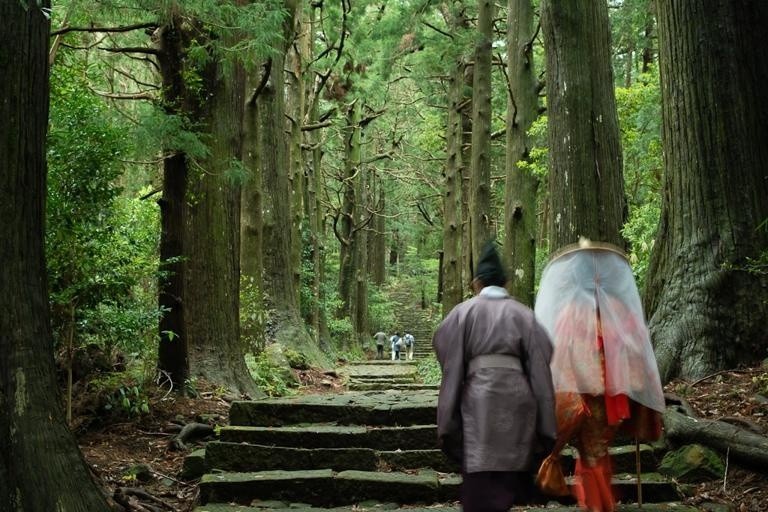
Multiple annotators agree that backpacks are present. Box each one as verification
[404,335,411,347]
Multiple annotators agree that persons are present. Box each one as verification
[431,239,554,512]
[390,329,402,362]
[531,241,663,511]
[373,329,387,358]
[403,330,415,360]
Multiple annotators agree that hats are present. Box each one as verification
[476,246,506,282]
